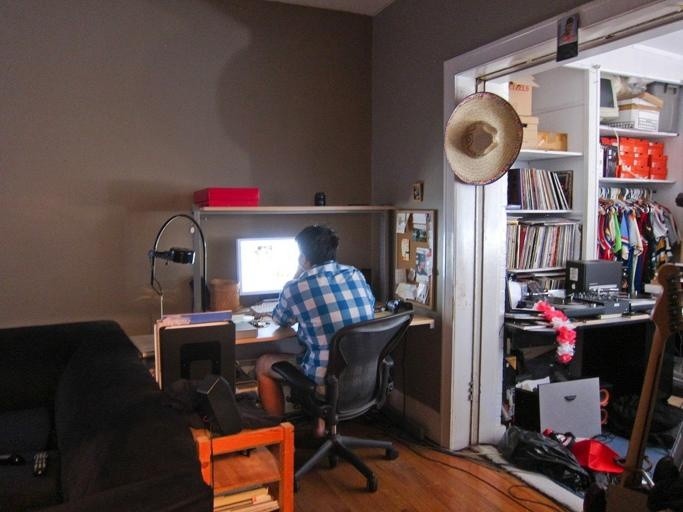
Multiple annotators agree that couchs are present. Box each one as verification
[0,320,211,511]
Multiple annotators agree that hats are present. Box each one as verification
[443,91,523,186]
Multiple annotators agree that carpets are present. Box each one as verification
[468,433,669,511]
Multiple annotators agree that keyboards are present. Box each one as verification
[250,302,279,314]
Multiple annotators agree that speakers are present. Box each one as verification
[196,373,242,436]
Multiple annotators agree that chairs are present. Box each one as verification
[272,311,413,492]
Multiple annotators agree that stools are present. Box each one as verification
[188,422,294,511]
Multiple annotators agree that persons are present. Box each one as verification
[255,222,377,422]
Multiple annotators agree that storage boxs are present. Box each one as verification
[600,97,660,131]
[508,76,567,152]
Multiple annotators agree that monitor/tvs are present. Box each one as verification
[236,236,301,297]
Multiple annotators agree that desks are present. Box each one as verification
[190,204,436,396]
[504,313,652,378]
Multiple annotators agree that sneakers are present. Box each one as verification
[303,430,327,450]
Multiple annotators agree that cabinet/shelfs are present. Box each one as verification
[506,43,682,273]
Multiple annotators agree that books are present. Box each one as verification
[509,164,585,297]
[214,484,279,512]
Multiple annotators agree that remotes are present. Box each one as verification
[32,449,50,477]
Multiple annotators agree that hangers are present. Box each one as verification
[599,185,664,217]
